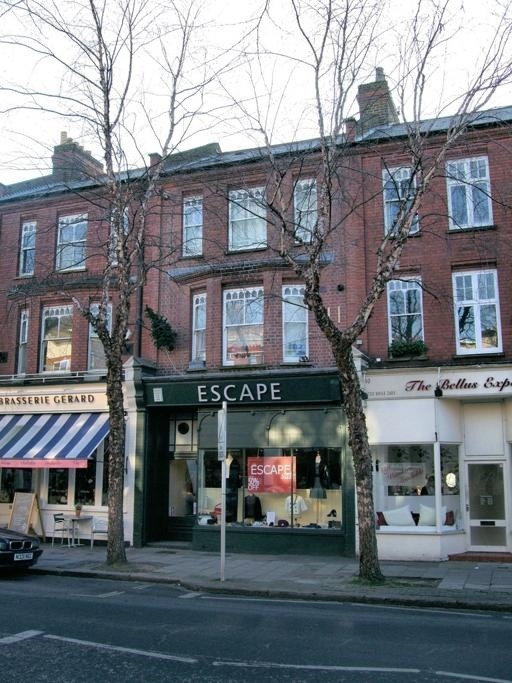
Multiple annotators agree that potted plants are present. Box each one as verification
[76,504,81,516]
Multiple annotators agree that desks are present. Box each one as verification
[55,514,94,547]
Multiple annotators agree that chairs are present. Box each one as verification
[51,512,79,549]
[90,519,109,551]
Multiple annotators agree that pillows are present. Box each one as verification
[381,503,416,527]
[417,502,447,526]
[375,508,412,526]
[412,510,454,527]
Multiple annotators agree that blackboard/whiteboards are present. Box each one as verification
[8,493,35,534]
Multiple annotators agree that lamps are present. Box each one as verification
[432,366,443,399]
[359,371,371,400]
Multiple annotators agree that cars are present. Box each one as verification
[0,465,87,504]
[0,527,44,578]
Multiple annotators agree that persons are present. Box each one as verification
[223,453,242,523]
[420,476,443,495]
